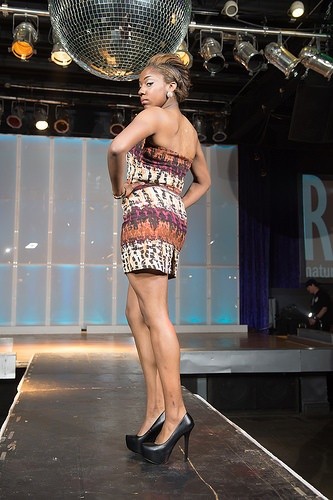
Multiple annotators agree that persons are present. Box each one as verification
[105,52,213,467]
[305,280,332,331]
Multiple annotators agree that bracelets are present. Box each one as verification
[113,187,126,200]
[313,316,321,321]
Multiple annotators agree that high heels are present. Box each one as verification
[125,410,195,465]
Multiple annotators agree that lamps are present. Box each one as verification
[11,13,39,60]
[48,27,72,66]
[54,105,73,133]
[6,100,27,128]
[175,32,193,69]
[198,29,226,73]
[287,1,305,23]
[233,30,265,74]
[221,1,238,17]
[211,114,228,142]
[266,33,301,79]
[298,37,333,79]
[192,113,208,142]
[32,102,50,131]
[109,107,125,136]
